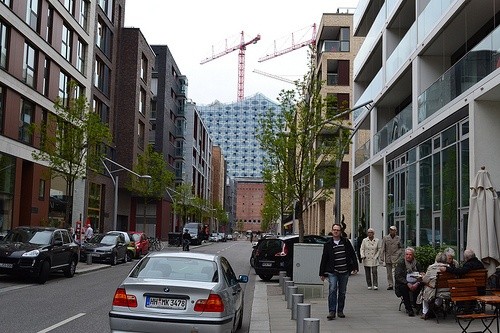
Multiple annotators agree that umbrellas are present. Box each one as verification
[465,166,500,278]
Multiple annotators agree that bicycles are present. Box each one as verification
[147,236,161,252]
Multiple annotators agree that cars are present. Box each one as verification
[108,251,249,333]
[208,230,277,249]
[129,232,150,259]
[79,232,128,265]
[104,231,137,261]
[49,189,67,212]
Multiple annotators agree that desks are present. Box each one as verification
[470,296,500,333]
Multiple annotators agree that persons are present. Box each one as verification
[84,224,94,242]
[318,223,359,320]
[182,229,193,251]
[379,226,402,290]
[395,247,423,317]
[444,247,459,269]
[419,252,450,320]
[456,249,489,320]
[359,228,380,290]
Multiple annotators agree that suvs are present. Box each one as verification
[251,233,328,282]
[0,226,80,284]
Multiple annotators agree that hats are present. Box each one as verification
[389,226,397,230]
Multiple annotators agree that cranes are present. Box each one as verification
[200,30,261,103]
[251,22,318,94]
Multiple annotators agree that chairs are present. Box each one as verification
[424,272,459,324]
[448,279,497,333]
[462,270,488,295]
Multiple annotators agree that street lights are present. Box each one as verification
[98,154,153,231]
[165,186,196,233]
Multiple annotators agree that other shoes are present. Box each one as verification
[387,287,393,290]
[421,314,425,319]
[367,286,372,290]
[408,311,414,316]
[373,285,379,290]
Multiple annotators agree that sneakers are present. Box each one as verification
[338,312,345,318]
[327,311,336,318]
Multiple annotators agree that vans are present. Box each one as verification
[182,222,203,246]
[413,228,446,248]
